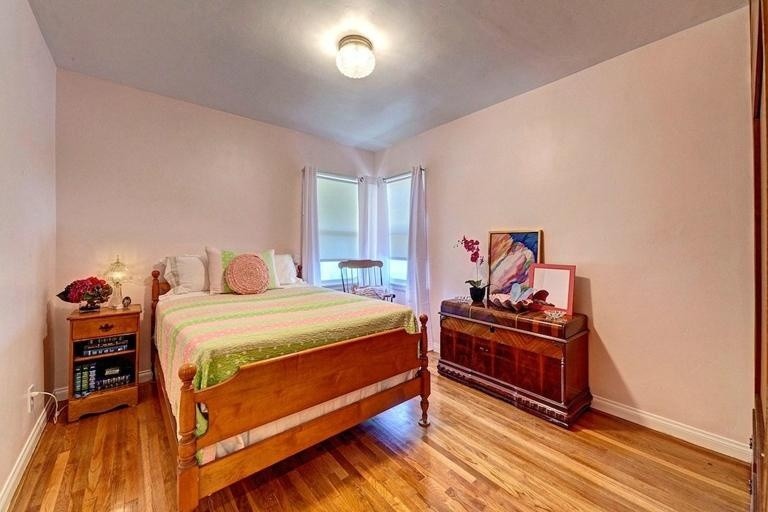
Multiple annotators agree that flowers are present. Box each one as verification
[454,237,500,290]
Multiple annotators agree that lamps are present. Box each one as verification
[103,255,132,309]
[337,34,375,79]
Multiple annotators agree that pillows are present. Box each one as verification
[206,245,283,295]
[275,254,298,284]
[225,253,271,295]
[160,254,210,294]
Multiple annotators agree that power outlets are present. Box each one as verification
[28,384,35,412]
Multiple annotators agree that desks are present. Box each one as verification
[437,297,593,428]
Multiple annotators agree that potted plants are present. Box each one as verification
[57,276,112,304]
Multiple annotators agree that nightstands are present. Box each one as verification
[66,304,141,422]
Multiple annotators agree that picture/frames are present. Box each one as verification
[528,263,576,316]
[487,229,541,301]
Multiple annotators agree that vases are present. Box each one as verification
[470,287,485,301]
[79,300,101,313]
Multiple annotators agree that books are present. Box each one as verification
[72,338,134,400]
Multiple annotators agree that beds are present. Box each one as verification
[152,265,430,512]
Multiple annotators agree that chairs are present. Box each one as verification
[338,259,395,302]
